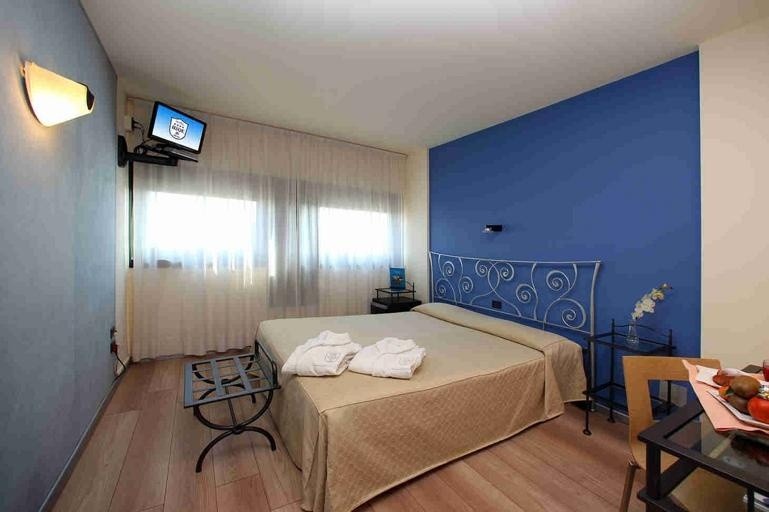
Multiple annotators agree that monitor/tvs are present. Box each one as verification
[148,101,208,154]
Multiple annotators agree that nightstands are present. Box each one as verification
[371,286,421,314]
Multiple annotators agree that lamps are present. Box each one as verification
[24,62,96,129]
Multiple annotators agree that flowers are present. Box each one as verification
[631,280,673,320]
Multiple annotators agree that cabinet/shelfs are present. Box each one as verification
[581,318,677,439]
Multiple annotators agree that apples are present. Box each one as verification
[747,396,769,424]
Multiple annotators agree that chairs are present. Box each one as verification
[619,351,755,511]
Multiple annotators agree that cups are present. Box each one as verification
[761,359,769,382]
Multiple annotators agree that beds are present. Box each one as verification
[254,250,603,512]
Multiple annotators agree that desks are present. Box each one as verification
[636,363,769,510]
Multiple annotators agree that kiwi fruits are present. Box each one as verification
[731,375,761,414]
